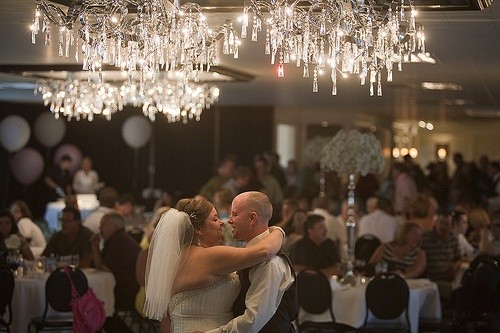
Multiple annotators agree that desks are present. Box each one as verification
[3,201,494,333]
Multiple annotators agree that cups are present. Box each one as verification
[14,253,80,278]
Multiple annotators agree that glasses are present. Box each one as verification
[59,217,74,223]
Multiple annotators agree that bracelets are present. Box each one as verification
[273,227,285,239]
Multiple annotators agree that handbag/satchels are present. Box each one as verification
[63,266,107,333]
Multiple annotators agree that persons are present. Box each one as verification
[198,146,283,203]
[285,159,403,243]
[451,219,500,333]
[288,214,343,278]
[193,191,299,333]
[0,209,35,262]
[91,212,142,333]
[366,222,427,278]
[118,193,134,215]
[9,201,46,256]
[142,198,283,333]
[40,154,72,207]
[408,193,490,280]
[82,185,122,233]
[392,154,500,212]
[41,207,94,267]
[73,156,104,194]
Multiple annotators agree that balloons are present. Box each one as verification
[0,111,82,184]
[121,116,153,148]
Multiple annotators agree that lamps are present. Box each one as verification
[317,128,386,286]
[21,0,426,158]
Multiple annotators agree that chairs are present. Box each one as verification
[102,272,158,333]
[294,268,356,333]
[357,272,411,333]
[0,262,15,333]
[27,265,88,333]
